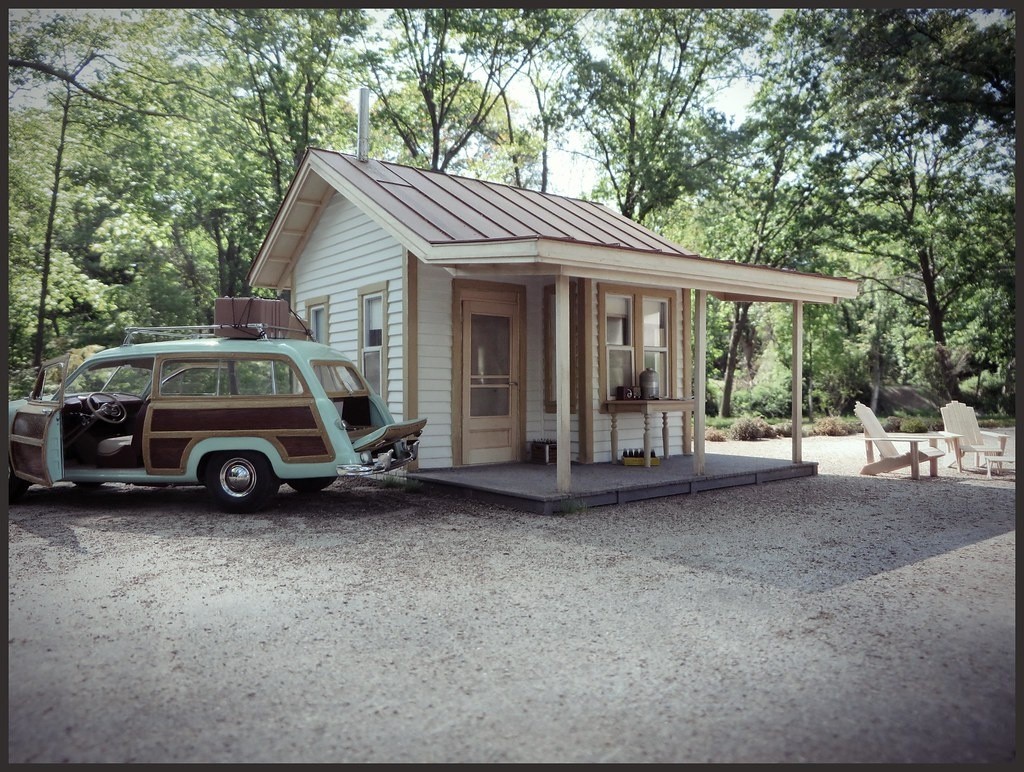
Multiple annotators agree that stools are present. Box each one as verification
[983,455,1015,479]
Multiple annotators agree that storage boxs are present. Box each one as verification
[622,455,659,466]
[531,442,558,466]
[213,297,289,338]
[289,314,309,340]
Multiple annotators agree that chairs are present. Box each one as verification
[854,401,948,480]
[95,398,151,469]
[940,399,1009,472]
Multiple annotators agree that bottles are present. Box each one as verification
[531,438,557,449]
[622,447,656,458]
[640,367,660,399]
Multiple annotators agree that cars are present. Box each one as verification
[7,323,427,506]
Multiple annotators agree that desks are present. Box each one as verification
[604,398,708,464]
